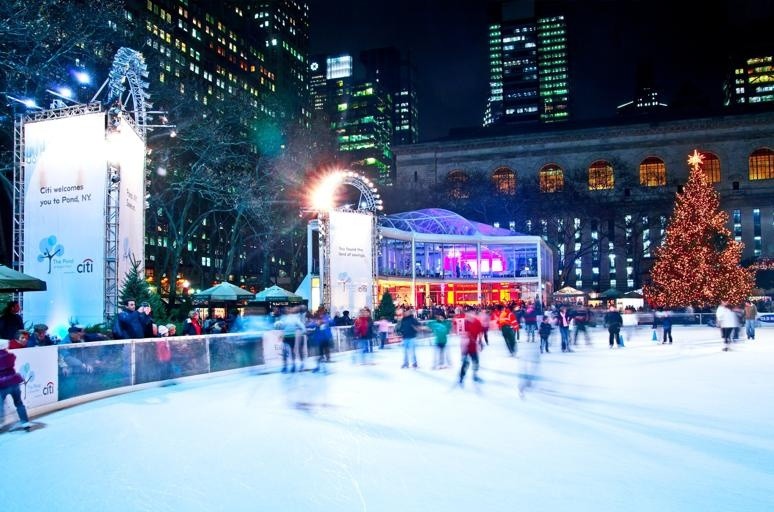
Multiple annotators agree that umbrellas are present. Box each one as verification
[0,263,47,293]
[193,281,256,301]
[248,283,302,303]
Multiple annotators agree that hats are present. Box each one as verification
[158,325,168,335]
[166,324,176,331]
[34,324,48,332]
[68,327,83,332]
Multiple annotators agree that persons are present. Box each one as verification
[456,261,472,279]
[1,297,758,435]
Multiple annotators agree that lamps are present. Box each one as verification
[108,46,177,138]
[343,169,383,215]
